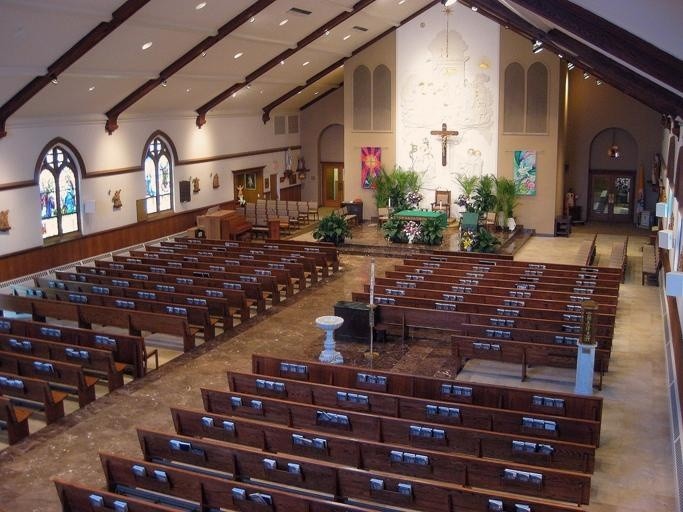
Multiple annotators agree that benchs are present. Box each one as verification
[0,232,662,511]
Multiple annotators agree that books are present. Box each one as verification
[2,319,118,389]
[375,256,599,352]
[0,235,322,319]
[88,362,565,511]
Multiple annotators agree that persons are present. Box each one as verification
[159,160,171,193]
[38,173,56,218]
[60,173,77,213]
[143,170,156,198]
[440,133,448,157]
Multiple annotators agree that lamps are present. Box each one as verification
[605,128,622,159]
[441,0,604,87]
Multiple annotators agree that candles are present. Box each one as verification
[439,200,442,211]
[388,196,390,209]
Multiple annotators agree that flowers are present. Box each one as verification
[453,194,470,207]
[400,220,422,235]
[459,230,476,253]
[407,192,423,203]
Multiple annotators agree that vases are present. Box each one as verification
[457,205,468,217]
[405,232,416,245]
[411,201,417,210]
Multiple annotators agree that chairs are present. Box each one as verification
[332,206,357,229]
[377,207,391,229]
[234,199,320,241]
[430,190,451,218]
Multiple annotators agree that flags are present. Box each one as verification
[635,164,646,215]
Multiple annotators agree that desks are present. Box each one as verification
[394,209,446,232]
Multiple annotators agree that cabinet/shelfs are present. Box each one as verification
[554,215,573,238]
[639,211,655,230]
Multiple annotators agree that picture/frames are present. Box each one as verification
[243,172,256,191]
[263,175,272,194]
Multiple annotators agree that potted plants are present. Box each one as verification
[476,173,530,231]
